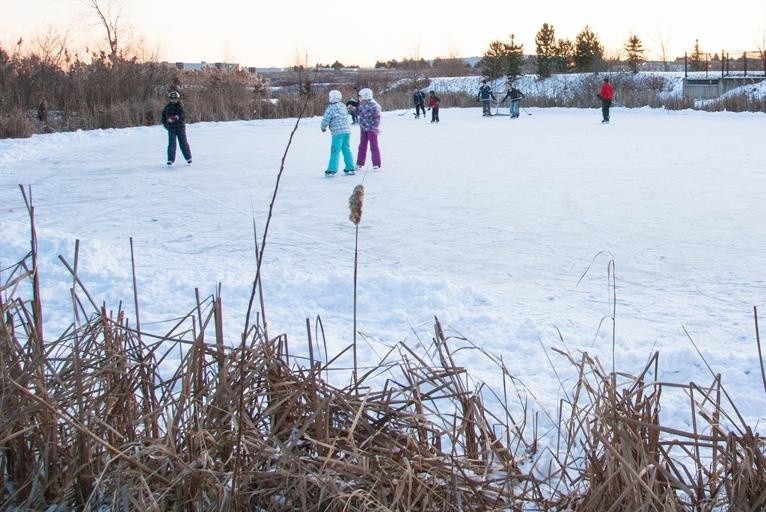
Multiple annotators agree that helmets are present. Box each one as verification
[328,90,342,104]
[358,88,372,100]
[168,92,179,99]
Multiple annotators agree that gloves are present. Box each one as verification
[167,115,179,123]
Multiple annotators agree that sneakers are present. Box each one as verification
[324,165,380,174]
[167,159,192,165]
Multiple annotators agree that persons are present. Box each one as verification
[500,83,526,118]
[161,91,193,166]
[428,90,440,122]
[596,78,614,123]
[413,88,427,118]
[318,90,357,174]
[476,80,497,117]
[345,96,360,124]
[354,87,382,170]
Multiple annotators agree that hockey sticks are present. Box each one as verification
[397,102,421,116]
[412,105,436,116]
